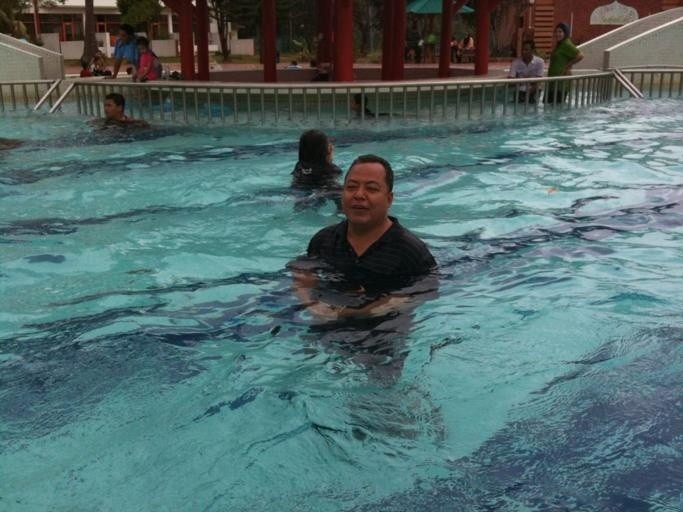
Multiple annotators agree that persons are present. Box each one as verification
[404,40,410,61]
[311,56,331,82]
[542,22,585,104]
[450,33,474,64]
[351,92,393,118]
[286,155,438,389]
[506,40,546,105]
[101,94,146,134]
[292,130,342,212]
[80,24,162,83]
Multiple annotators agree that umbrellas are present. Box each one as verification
[404,0,476,15]
